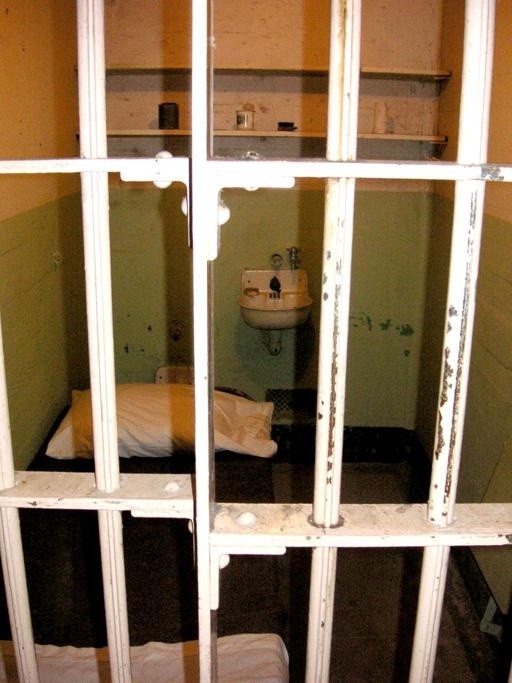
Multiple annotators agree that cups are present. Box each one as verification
[158,103,179,130]
[236,110,255,131]
[277,121,293,131]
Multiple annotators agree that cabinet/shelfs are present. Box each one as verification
[102,59,453,145]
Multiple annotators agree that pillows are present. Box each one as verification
[46,387,279,461]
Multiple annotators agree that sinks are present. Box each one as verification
[238,292,314,330]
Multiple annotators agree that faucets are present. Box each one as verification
[267,279,284,297]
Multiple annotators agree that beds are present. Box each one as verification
[2,386,287,682]
